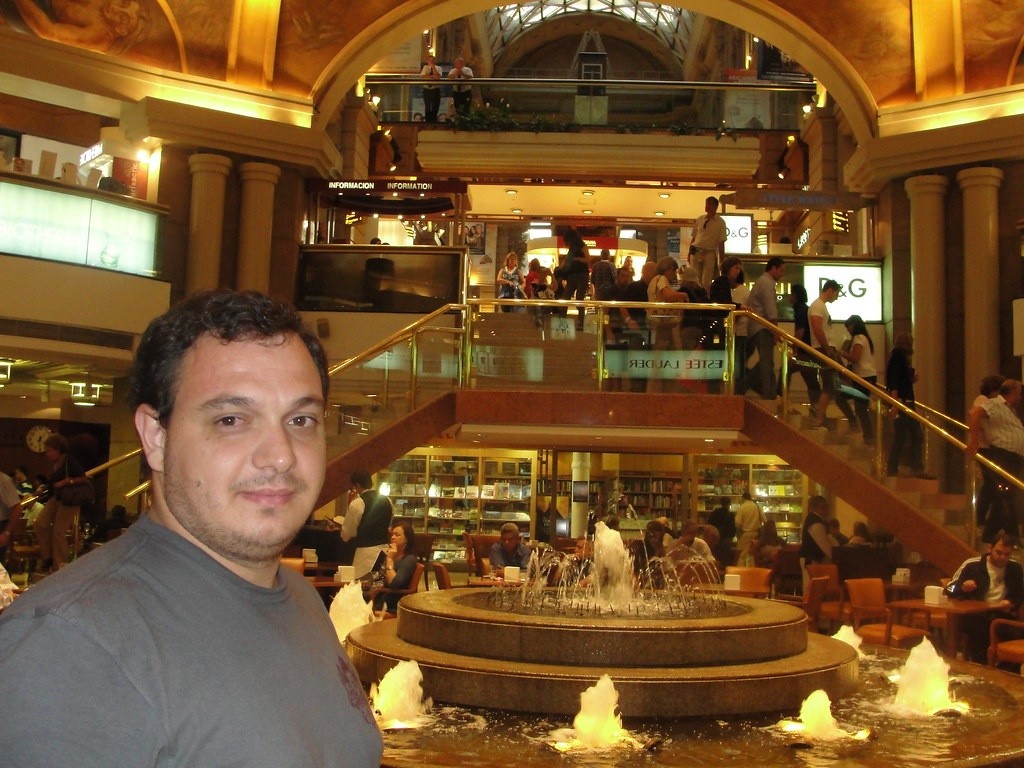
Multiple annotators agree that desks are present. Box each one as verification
[470,576,524,588]
[889,597,1004,659]
[688,582,770,599]
[306,576,371,610]
[303,561,342,577]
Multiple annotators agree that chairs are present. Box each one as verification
[674,560,1024,675]
[279,532,579,629]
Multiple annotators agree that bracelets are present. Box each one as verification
[3,531,11,536]
[70,478,73,485]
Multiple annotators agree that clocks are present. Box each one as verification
[26,425,53,453]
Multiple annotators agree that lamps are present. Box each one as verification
[69,380,102,407]
[0,359,16,387]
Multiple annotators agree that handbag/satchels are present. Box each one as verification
[559,254,573,276]
[645,274,685,329]
[513,283,529,300]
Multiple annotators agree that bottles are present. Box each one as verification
[305,557,319,582]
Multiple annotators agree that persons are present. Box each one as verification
[342,196,1022,671]
[35,475,48,504]
[36,434,87,573]
[421,56,441,122]
[438,113,447,123]
[84,505,130,550]
[0,290,385,768]
[414,113,423,123]
[0,472,21,564]
[14,467,33,518]
[447,58,474,117]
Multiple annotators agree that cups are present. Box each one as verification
[333,574,341,582]
[488,569,497,579]
[940,595,949,604]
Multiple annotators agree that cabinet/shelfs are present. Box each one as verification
[374,446,538,573]
[689,454,808,546]
[590,469,682,521]
